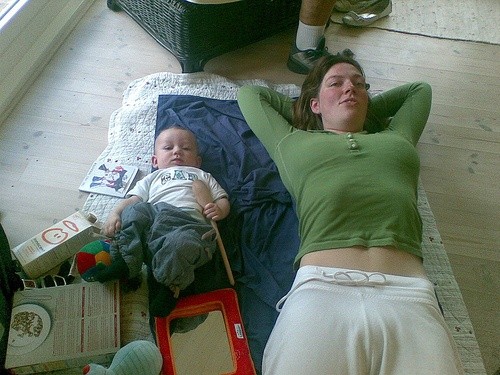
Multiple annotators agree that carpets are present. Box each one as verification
[331,0,500,45]
[46,73,488,375]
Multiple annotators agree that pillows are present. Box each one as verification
[28,255,155,375]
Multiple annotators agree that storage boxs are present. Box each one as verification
[5,280,121,375]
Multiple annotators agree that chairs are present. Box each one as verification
[108,0,301,74]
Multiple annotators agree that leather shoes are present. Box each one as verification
[335,0,378,12]
[342,0,392,26]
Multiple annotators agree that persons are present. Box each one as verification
[287,0,337,73]
[97,127,229,319]
[236,53,464,375]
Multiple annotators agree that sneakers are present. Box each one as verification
[287,35,332,74]
